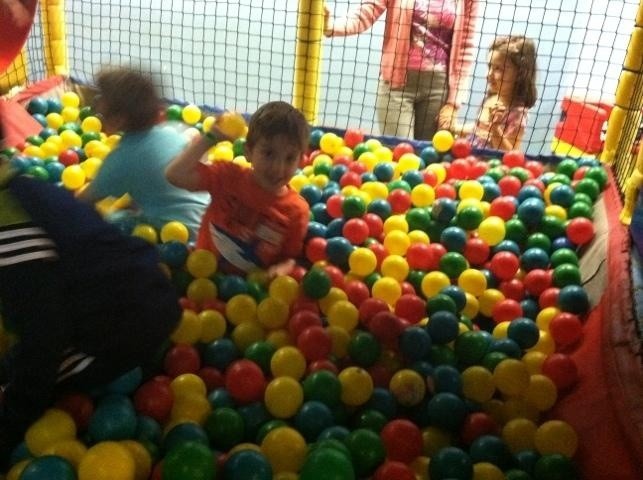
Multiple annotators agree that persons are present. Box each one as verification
[436,34,541,151]
[165,100,311,288]
[0,1,184,464]
[323,0,478,142]
[70,62,211,244]
